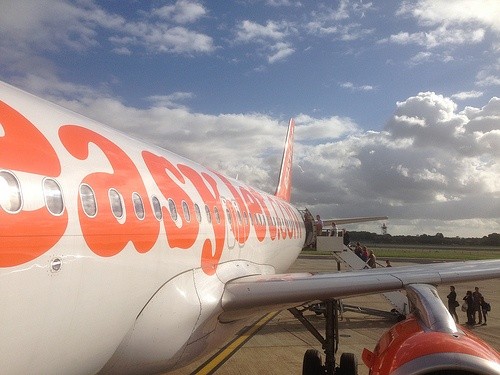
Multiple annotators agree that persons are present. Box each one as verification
[463,287,487,326]
[447,286,459,323]
[354,241,392,268]
[315,215,350,246]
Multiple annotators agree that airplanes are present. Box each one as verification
[0,79,500,375]
